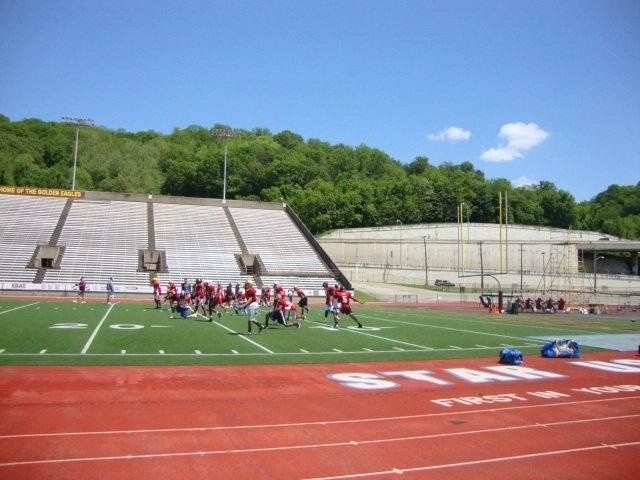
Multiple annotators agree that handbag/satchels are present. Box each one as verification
[498,347,523,365]
[541,338,580,358]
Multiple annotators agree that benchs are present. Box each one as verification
[2,193,341,296]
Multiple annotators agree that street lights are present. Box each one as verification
[593,256,606,294]
[397,219,403,228]
[422,235,429,288]
[58,116,98,191]
[541,252,546,295]
[210,127,244,206]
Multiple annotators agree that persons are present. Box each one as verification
[106,277,115,305]
[151,278,161,309]
[79,277,87,303]
[163,278,363,334]
[514,297,566,314]
